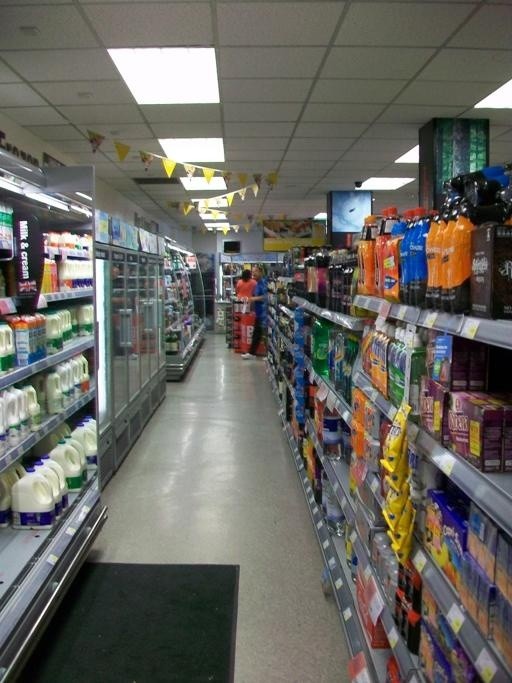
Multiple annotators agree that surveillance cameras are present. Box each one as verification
[355,181,362,187]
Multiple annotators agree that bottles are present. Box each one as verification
[305,196,472,600]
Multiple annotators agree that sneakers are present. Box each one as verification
[262,357,267,361]
[241,353,256,360]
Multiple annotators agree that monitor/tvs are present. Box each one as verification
[222,239,241,255]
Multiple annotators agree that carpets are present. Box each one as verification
[16,560,240,683]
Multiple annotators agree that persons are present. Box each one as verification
[233,267,256,296]
[241,264,269,361]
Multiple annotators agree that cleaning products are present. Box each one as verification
[0,299,97,531]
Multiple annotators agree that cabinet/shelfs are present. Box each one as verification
[225,310,232,344]
[0,140,205,683]
[219,259,265,303]
[262,278,512,683]
